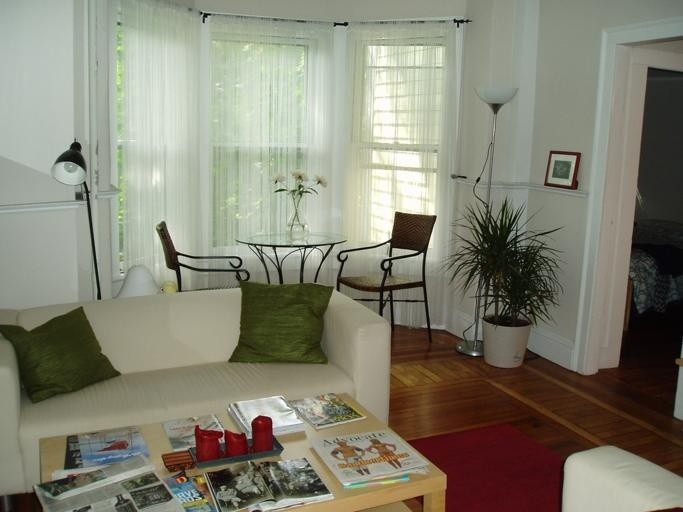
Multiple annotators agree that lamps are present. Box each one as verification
[454,68,521,356]
[50,139,103,302]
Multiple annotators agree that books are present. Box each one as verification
[162,470,217,512]
[311,428,429,488]
[161,412,224,450]
[203,457,335,512]
[289,394,368,429]
[226,394,305,440]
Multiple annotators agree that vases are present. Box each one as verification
[284,206,309,242]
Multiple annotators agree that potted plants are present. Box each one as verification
[437,194,573,371]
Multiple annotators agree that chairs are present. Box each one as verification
[154,220,249,292]
[332,210,437,353]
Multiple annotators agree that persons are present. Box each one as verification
[365,439,402,469]
[216,466,323,510]
[330,440,371,475]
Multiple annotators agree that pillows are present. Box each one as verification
[226,281,334,363]
[0,305,120,403]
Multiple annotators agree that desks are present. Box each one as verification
[34,388,448,512]
[232,233,347,286]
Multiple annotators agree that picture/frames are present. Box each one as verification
[542,150,582,189]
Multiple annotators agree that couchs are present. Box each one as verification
[0,280,395,512]
[559,442,681,512]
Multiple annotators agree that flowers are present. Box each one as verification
[268,168,327,230]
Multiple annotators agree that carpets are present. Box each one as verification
[1,412,597,512]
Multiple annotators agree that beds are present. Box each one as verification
[621,187,682,336]
